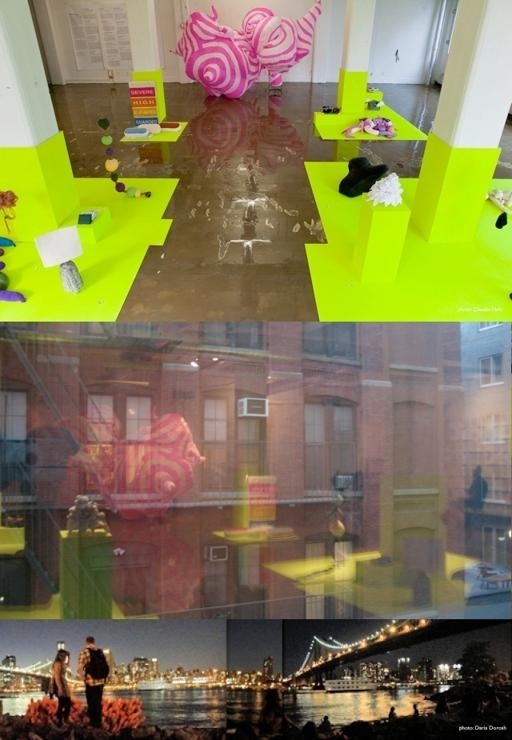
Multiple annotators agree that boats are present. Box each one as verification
[323,678,379,693]
[137,679,164,691]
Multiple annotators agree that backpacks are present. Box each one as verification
[83,648,109,683]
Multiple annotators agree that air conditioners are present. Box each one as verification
[236,396,270,419]
[202,543,230,564]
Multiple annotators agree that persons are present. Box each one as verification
[76,635,105,728]
[49,648,74,727]
[464,463,490,511]
